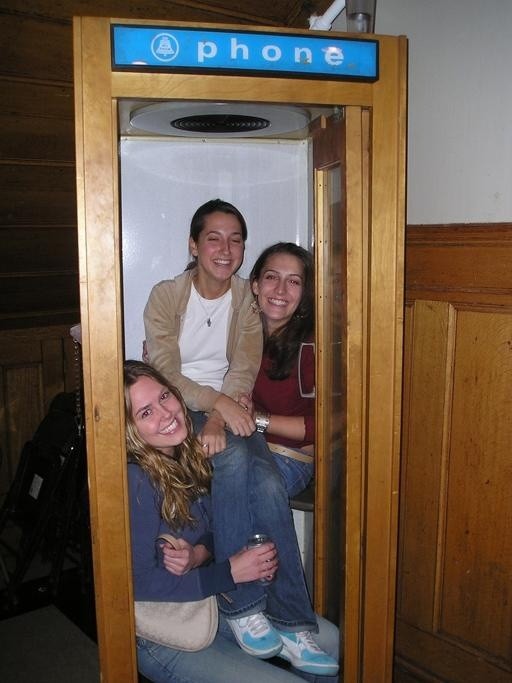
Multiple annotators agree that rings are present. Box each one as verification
[244,404,247,411]
[199,442,209,447]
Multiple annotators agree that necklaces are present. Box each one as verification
[196,282,224,326]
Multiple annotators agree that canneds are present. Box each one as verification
[248,534,272,586]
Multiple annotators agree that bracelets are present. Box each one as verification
[256,410,272,433]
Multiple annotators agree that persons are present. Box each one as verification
[122,358,341,683]
[140,196,340,677]
[233,240,316,502]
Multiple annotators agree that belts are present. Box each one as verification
[268,442,314,464]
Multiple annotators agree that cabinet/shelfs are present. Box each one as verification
[2,72,79,318]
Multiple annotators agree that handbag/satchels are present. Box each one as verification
[135,597,219,654]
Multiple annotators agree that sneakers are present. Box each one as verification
[277,630,340,679]
[226,612,283,660]
